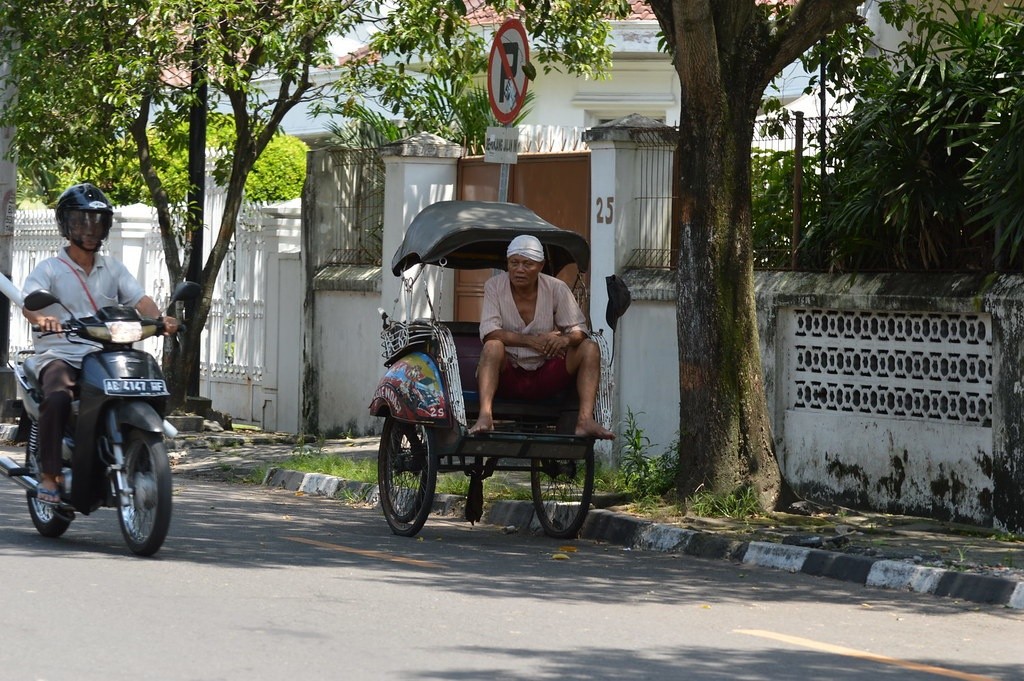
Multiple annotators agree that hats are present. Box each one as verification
[606,274,632,332]
[506,235,544,263]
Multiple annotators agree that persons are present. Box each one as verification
[464,234,617,441]
[20,182,178,506]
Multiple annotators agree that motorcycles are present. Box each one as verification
[0,286,205,558]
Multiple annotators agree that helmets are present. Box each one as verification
[55,183,114,252]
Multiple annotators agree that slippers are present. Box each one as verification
[37,483,60,505]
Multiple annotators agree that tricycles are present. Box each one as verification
[367,198,612,535]
